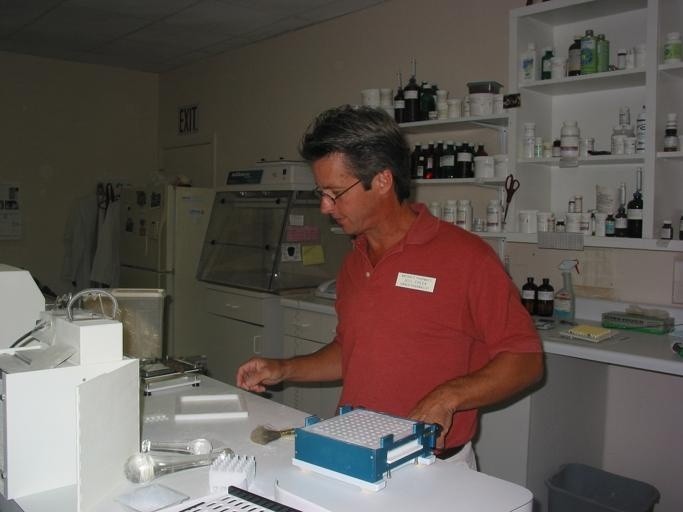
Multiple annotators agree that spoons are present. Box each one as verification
[124,447,235,483]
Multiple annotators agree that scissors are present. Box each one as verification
[503,173,520,224]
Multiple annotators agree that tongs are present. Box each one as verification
[141,437,214,458]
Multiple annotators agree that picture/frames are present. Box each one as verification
[172,100,202,141]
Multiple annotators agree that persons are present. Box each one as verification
[234,103,548,472]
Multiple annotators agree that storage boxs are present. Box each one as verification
[84,280,165,365]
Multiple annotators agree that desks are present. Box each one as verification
[0,360,535,512]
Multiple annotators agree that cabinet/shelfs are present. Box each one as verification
[475,304,682,507]
[504,0,683,310]
[279,293,341,420]
[375,110,512,240]
[203,286,277,388]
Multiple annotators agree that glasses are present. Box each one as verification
[312,178,364,210]
